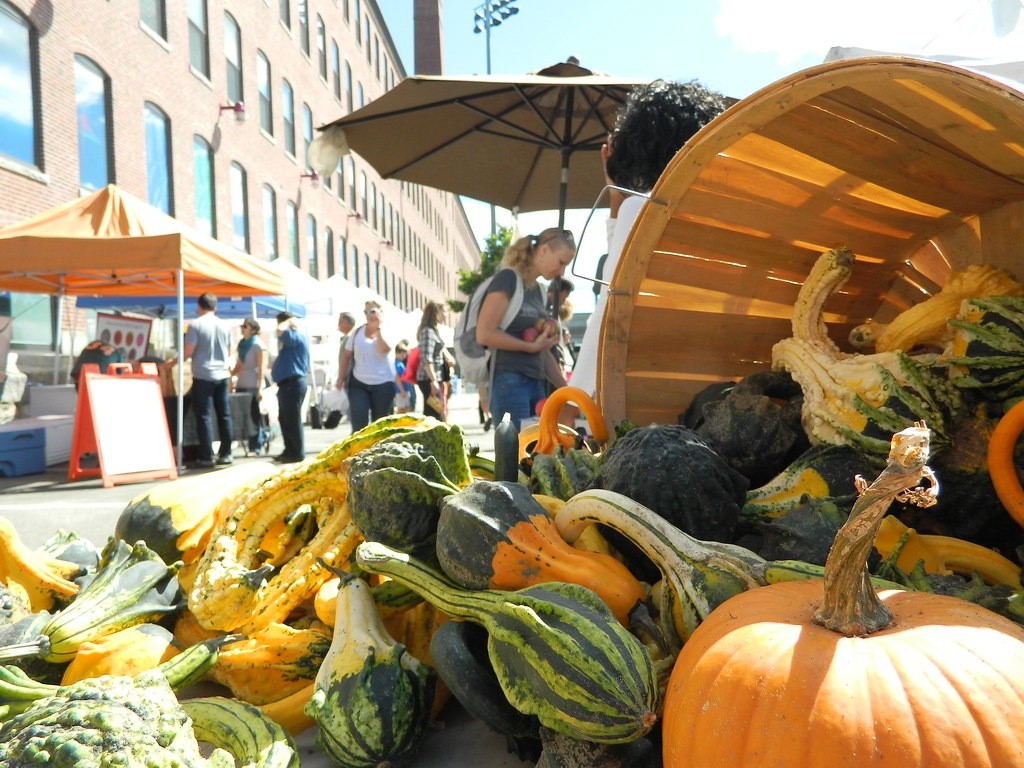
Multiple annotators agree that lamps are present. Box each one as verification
[301,170,320,189]
[380,240,392,249]
[348,212,362,226]
[219,102,247,125]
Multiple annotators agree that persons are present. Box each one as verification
[271,311,310,464]
[550,78,731,459]
[337,301,456,440]
[71,341,122,400]
[477,228,581,468]
[153,291,233,469]
[229,319,268,458]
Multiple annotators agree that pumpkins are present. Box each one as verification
[0,248,1024,768]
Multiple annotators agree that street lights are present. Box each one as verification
[474,1,520,240]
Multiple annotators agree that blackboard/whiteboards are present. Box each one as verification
[68,363,178,488]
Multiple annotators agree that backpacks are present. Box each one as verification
[453,268,548,382]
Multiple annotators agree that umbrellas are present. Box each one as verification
[316,63,743,318]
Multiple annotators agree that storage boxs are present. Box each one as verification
[0,413,74,478]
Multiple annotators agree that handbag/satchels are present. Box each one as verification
[400,345,420,384]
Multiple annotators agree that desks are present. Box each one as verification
[163,391,260,465]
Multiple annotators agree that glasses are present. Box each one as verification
[542,229,573,243]
[240,325,252,329]
[364,310,376,314]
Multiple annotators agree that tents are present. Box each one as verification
[0,183,461,466]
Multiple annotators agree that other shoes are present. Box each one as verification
[186,459,215,469]
[216,454,233,464]
[246,451,257,457]
[262,433,270,453]
[274,452,303,463]
